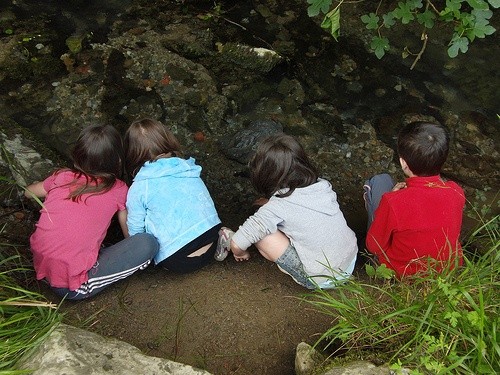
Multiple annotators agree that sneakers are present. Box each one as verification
[214,227,235,261]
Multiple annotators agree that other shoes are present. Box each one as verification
[238,253,274,267]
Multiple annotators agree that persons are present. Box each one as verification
[363,120,466,281]
[123,118,234,274]
[230,133,358,290]
[25,122,160,301]
